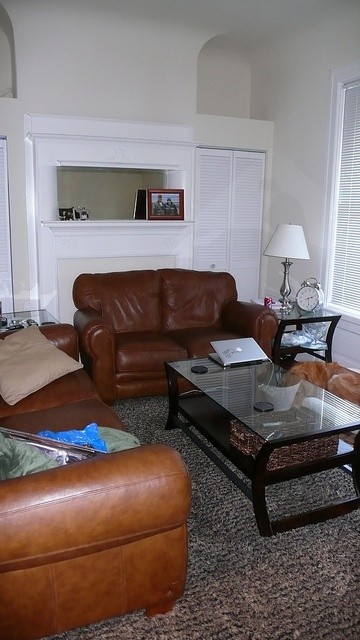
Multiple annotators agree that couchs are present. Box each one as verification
[72,268,279,406]
[0,323,192,640]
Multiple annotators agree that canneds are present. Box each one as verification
[264,296,272,308]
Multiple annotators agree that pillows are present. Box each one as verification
[0,324,85,406]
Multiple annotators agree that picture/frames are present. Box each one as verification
[0,425,95,466]
[145,188,184,220]
[58,207,76,221]
[133,189,145,219]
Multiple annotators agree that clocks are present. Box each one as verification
[295,277,325,312]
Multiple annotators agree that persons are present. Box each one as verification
[165,198,179,216]
[152,195,166,215]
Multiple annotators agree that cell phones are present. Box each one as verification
[26,318,38,326]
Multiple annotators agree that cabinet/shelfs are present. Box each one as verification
[193,148,266,302]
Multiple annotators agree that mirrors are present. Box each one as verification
[56,166,167,221]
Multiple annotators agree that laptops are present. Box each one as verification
[208,337,268,367]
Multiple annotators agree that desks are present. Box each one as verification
[262,223,311,309]
